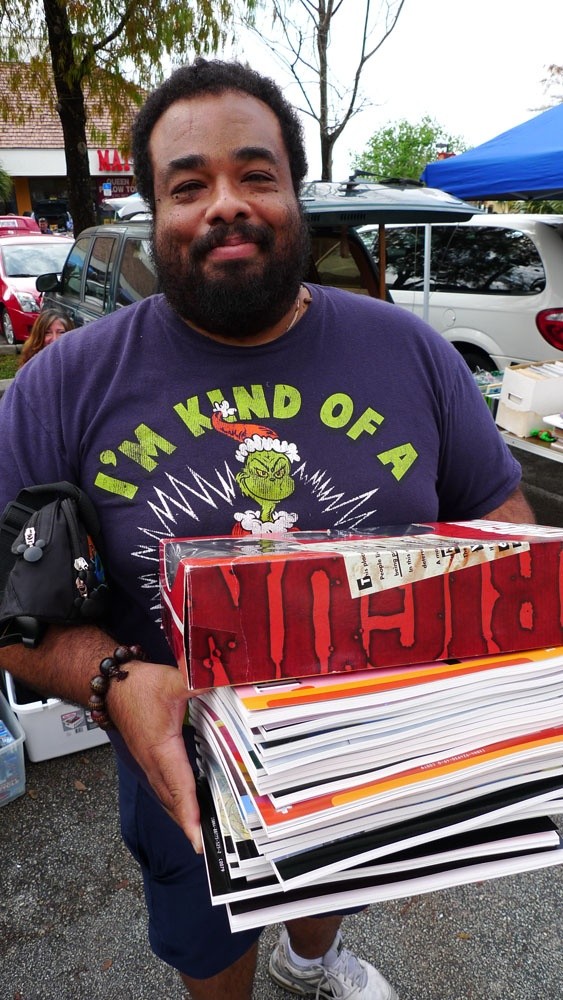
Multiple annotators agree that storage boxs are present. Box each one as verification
[4,669,110,763]
[0,691,27,808]
[494,360,563,437]
[158,519,563,692]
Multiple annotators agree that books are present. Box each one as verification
[185,646,563,934]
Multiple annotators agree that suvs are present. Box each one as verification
[35,180,563,374]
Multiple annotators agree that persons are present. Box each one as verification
[7,211,73,371]
[0,60,541,1000]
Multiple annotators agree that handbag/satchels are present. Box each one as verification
[0,484,115,649]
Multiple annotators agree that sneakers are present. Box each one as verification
[270,928,399,1000]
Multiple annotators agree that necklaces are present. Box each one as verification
[288,297,302,331]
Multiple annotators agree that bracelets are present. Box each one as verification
[88,644,149,730]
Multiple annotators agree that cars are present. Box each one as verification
[0,216,77,346]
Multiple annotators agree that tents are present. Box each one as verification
[101,192,152,223]
[424,104,563,327]
